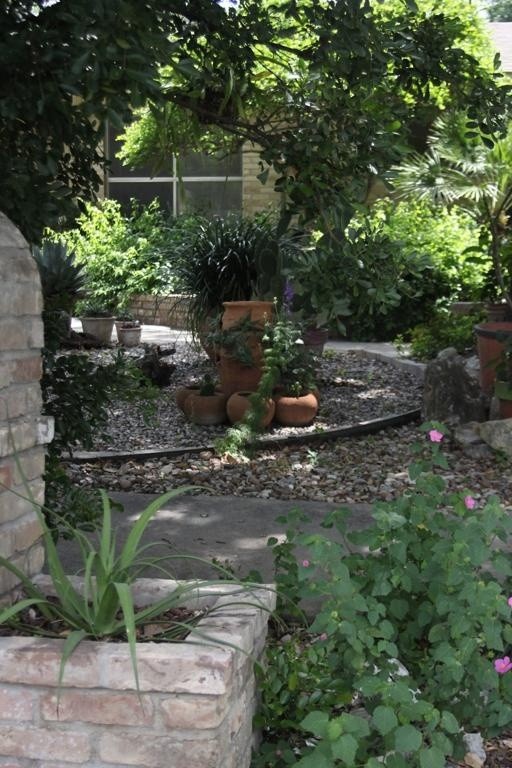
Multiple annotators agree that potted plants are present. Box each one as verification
[46,196,330,451]
[387,150,512,418]
[0,419,279,768]
